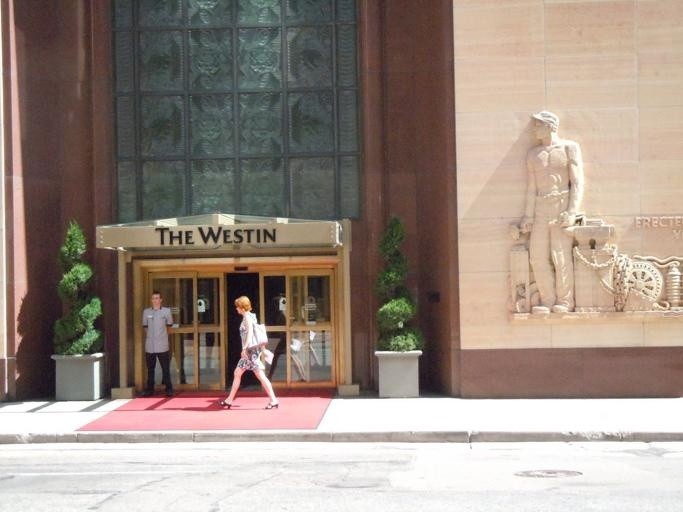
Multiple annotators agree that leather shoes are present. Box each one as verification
[166,389,172,397]
[142,390,153,396]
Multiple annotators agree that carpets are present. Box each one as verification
[74,387,336,433]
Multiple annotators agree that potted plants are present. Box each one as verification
[47,217,110,403]
[369,212,425,401]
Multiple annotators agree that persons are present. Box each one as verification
[218,295,278,409]
[519,110,583,314]
[140,290,174,396]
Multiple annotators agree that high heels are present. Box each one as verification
[219,400,232,409]
[265,403,279,408]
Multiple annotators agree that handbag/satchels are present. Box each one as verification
[242,324,268,351]
